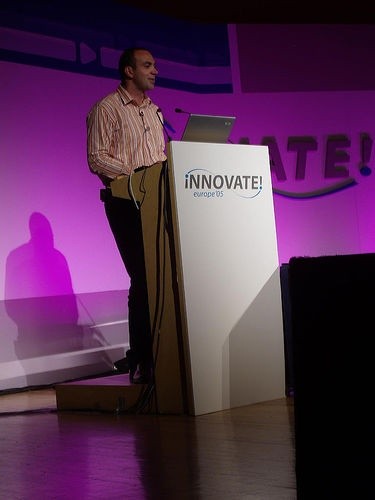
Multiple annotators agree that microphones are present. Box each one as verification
[175,108,191,114]
[156,108,171,142]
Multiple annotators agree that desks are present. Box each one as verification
[287,254,375,466]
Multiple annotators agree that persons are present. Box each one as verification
[85,46,166,384]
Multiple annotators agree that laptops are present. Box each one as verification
[181,115,236,143]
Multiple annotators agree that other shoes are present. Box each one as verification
[128,363,152,384]
